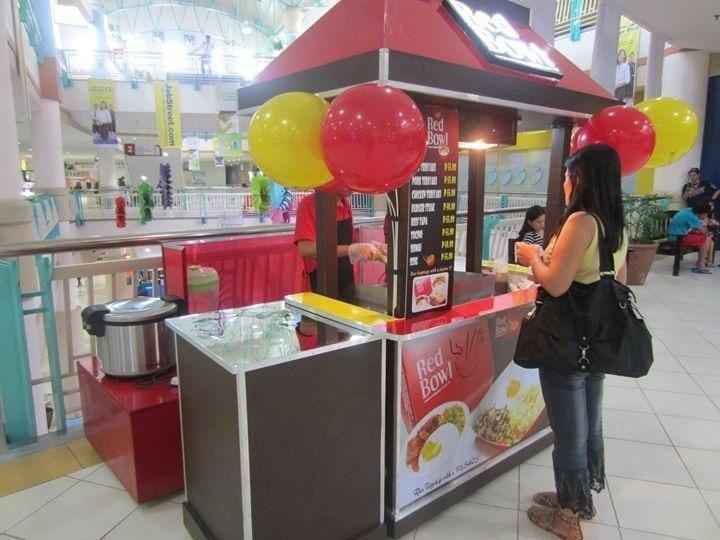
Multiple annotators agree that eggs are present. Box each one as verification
[417,423,460,472]
[494,367,537,410]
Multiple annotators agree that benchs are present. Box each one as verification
[648,210,716,276]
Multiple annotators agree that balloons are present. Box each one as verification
[572,106,656,176]
[635,97,698,167]
[320,84,427,195]
[248,92,334,188]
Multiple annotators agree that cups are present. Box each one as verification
[416,295,431,308]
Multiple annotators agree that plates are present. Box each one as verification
[404,400,470,476]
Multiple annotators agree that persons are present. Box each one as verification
[670,199,718,274]
[518,205,546,247]
[69,176,127,206]
[615,50,635,99]
[216,111,235,132]
[280,187,292,223]
[682,168,720,265]
[93,101,115,139]
[515,143,627,540]
[295,315,351,350]
[294,187,388,290]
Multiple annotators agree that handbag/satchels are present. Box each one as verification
[514,212,652,378]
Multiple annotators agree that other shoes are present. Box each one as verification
[705,263,714,267]
[691,267,714,274]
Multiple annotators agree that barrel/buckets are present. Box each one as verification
[187,264,220,315]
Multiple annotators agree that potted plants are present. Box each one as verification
[621,192,671,285]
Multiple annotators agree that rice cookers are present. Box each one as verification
[82,293,186,379]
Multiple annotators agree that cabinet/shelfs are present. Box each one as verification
[76,354,184,504]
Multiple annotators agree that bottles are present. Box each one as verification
[495,270,510,296]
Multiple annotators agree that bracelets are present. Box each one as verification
[530,257,541,270]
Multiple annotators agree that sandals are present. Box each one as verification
[533,491,596,519]
[528,505,583,540]
